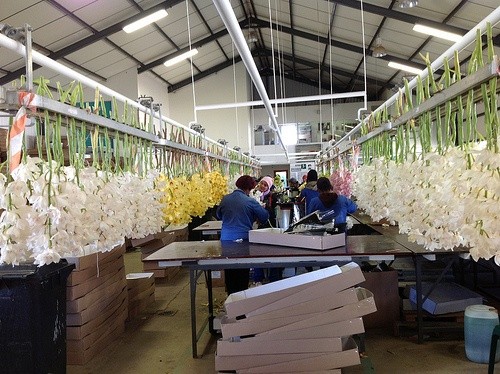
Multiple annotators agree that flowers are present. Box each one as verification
[0,75,259,264]
[311,21,500,266]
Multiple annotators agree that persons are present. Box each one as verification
[300,172,320,207]
[216,175,268,296]
[307,177,357,224]
[247,176,274,228]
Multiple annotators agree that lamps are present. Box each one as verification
[248,27,258,43]
[120,7,169,35]
[372,34,388,57]
[397,0,419,9]
[162,46,198,67]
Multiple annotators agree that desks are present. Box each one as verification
[368,223,489,343]
[348,208,388,224]
[193,221,227,241]
[142,235,412,359]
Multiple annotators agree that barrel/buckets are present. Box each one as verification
[464,305,500,363]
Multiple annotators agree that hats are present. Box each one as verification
[252,176,275,192]
[306,169,318,182]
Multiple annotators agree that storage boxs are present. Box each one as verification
[64,228,483,374]
